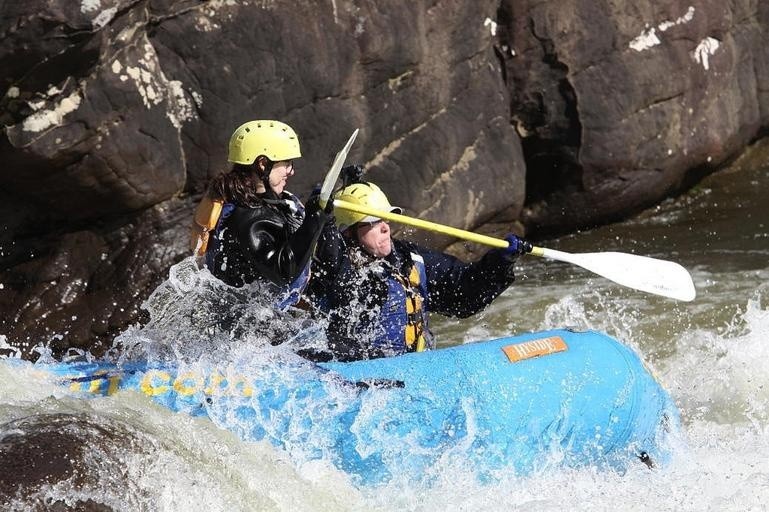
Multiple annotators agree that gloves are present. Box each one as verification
[492,233,533,262]
[341,165,364,186]
[305,188,334,235]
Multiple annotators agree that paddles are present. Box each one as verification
[317,129,359,212]
[333,200,696,303]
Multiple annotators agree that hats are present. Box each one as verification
[360,205,402,222]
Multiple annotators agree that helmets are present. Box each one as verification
[228,119,302,164]
[333,182,403,234]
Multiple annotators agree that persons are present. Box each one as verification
[191,118,367,367]
[315,179,535,364]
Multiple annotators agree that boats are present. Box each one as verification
[1,315,680,496]
